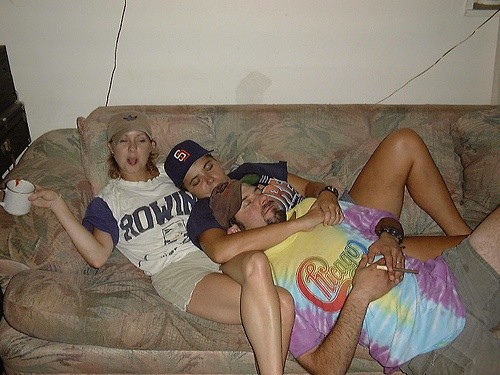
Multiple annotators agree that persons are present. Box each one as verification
[208,173,500,375]
[28,110,296,375]
[164,128,474,263]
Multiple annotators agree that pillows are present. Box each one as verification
[332,109,500,236]
[75,114,217,200]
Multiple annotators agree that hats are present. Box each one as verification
[107,111,152,145]
[164,139,214,188]
[209,174,259,228]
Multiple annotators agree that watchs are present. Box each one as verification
[378,226,402,243]
[318,186,339,197]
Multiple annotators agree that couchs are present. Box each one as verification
[0,105,500,375]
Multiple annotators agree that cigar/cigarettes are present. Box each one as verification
[376,265,419,274]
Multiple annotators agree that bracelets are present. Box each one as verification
[49,192,62,209]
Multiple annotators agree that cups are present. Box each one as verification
[0,179,36,216]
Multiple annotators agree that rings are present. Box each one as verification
[336,212,340,214]
[397,261,402,263]
